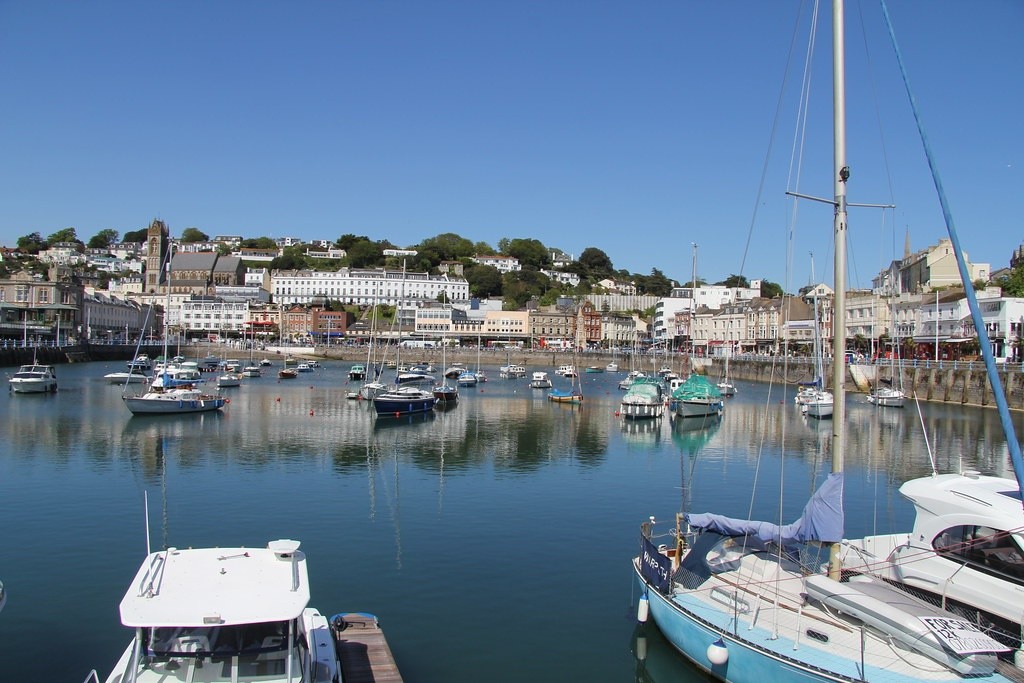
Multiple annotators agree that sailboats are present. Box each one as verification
[431,328,458,403]
[715,335,738,396]
[120,238,227,415]
[9,327,59,394]
[625,1,1024,683]
[794,254,834,419]
[617,316,687,391]
[372,258,435,416]
[474,319,486,383]
[359,307,399,400]
[547,326,584,403]
[103,312,320,391]
[865,286,906,408]
[499,319,518,379]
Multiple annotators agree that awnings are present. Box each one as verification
[692,339,735,347]
[945,338,973,342]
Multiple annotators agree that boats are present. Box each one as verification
[531,372,552,388]
[586,365,604,373]
[370,359,439,384]
[80,489,348,683]
[457,367,477,388]
[349,363,367,381]
[442,362,468,379]
[499,364,526,373]
[605,363,620,373]
[554,363,578,378]
[822,387,1024,631]
[215,373,242,387]
[620,376,665,419]
[671,370,725,418]
